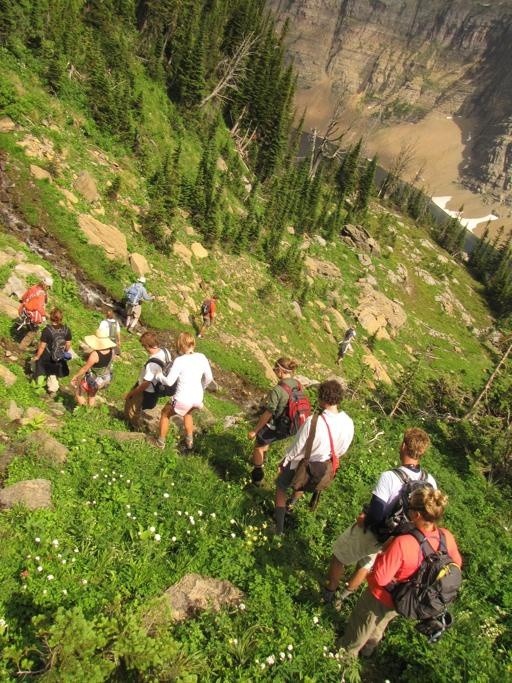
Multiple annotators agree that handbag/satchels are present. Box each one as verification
[288,457,333,493]
[30,310,43,324]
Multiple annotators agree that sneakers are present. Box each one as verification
[155,436,167,450]
[126,325,134,334]
[184,437,194,450]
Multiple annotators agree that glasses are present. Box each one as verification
[272,367,281,371]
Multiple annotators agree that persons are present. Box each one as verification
[197,294,220,340]
[16,275,52,351]
[311,425,441,617]
[331,480,467,663]
[104,308,121,357]
[143,328,215,451]
[27,304,73,399]
[261,378,357,538]
[119,331,174,433]
[122,275,156,334]
[68,325,118,407]
[243,353,314,492]
[335,337,355,366]
[342,323,357,343]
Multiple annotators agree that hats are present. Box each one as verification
[42,276,53,285]
[137,276,146,282]
[84,327,116,350]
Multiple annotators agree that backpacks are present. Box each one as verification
[378,468,428,535]
[143,346,178,398]
[391,527,462,620]
[276,379,311,440]
[126,282,140,303]
[200,303,209,314]
[45,323,69,362]
